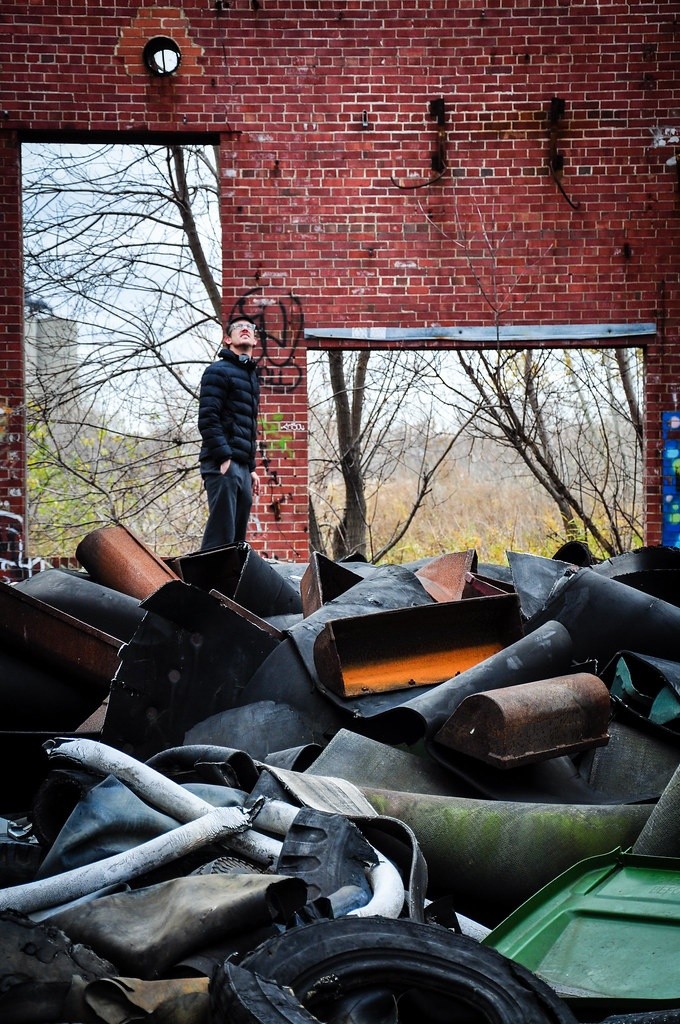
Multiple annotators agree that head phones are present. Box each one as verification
[232,352,249,365]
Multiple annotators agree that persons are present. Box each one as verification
[199,316,262,549]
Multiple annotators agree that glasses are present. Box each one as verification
[228,323,256,335]
